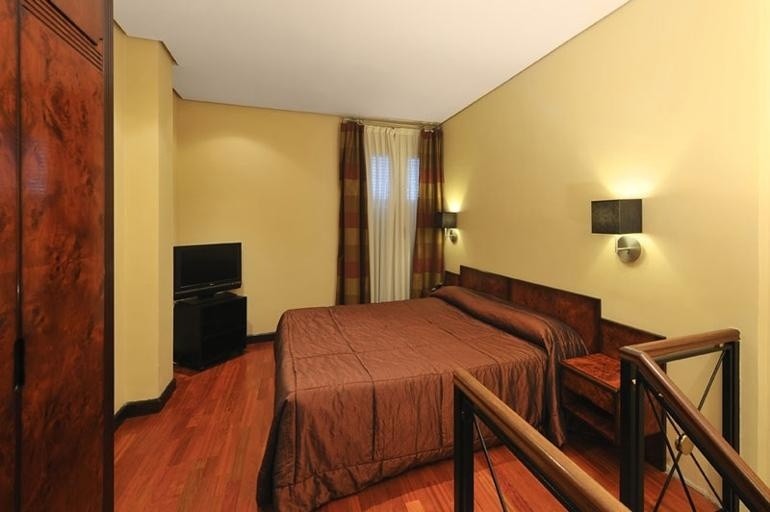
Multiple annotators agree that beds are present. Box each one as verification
[258,264,601,512]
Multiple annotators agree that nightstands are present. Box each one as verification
[422,271,459,296]
[560,317,666,472]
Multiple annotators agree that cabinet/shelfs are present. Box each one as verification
[173,292,248,373]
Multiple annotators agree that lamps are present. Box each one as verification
[433,212,459,243]
[591,200,643,264]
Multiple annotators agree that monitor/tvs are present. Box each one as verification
[173,241,243,299]
